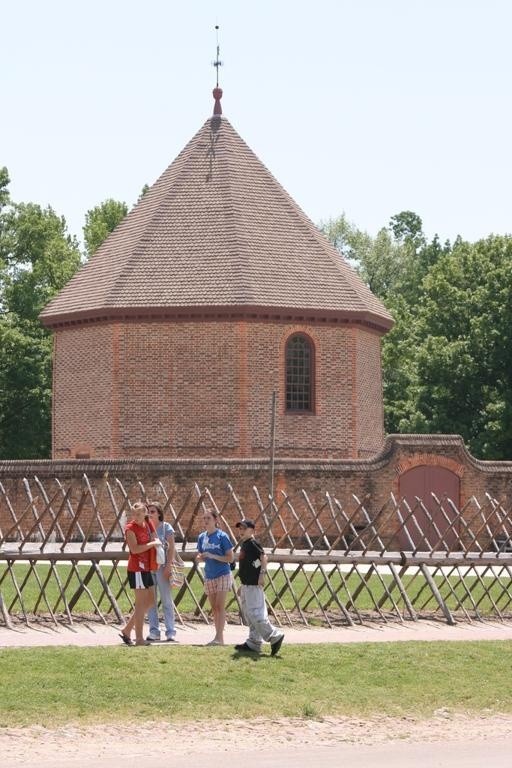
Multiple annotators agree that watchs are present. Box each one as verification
[259,568,265,575]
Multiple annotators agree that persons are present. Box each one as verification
[147,502,178,642]
[195,508,235,645]
[231,517,285,655]
[119,500,165,647]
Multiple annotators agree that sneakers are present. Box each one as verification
[147,633,161,641]
[271,635,284,657]
[234,642,252,651]
[167,633,176,641]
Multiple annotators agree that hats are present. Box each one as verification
[235,518,256,530]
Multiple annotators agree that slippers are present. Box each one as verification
[206,640,226,646]
[118,633,150,646]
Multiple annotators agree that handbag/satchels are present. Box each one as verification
[149,548,160,574]
[168,551,186,589]
